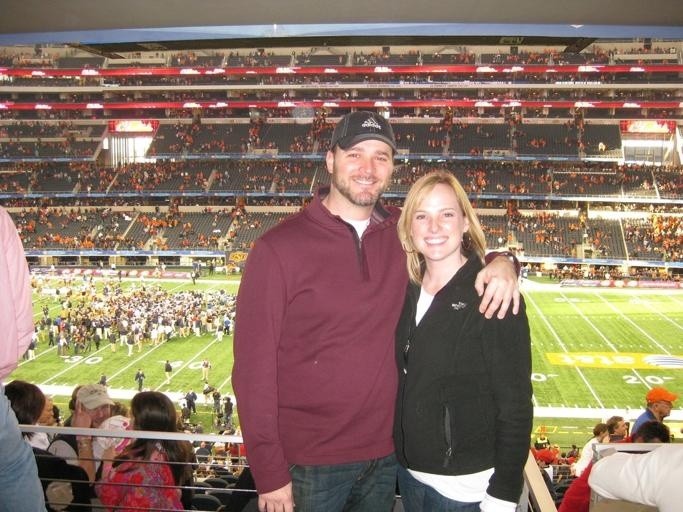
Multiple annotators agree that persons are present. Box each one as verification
[0,208,47,511]
[220,109,522,511]
[4,284,250,510]
[0,45,683,283]
[390,168,536,511]
[538,390,683,512]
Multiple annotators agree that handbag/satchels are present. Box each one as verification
[96,415,131,451]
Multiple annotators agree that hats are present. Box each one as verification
[645,388,676,403]
[330,111,399,155]
[76,383,116,409]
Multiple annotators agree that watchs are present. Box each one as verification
[502,253,518,267]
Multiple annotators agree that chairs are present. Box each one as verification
[554,477,574,510]
[189,474,238,512]
